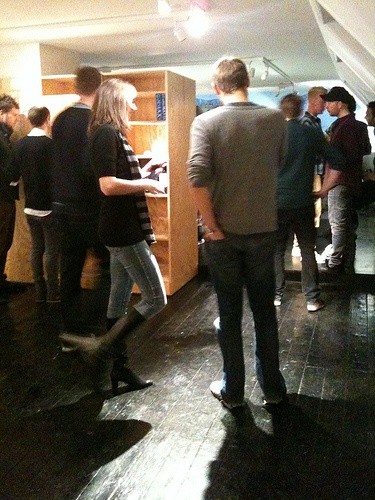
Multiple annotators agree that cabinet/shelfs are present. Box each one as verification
[38,69,198,298]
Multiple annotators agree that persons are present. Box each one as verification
[195,84,375,329]
[186,54,290,411]
[0,66,170,390]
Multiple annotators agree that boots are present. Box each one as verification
[34,300,61,317]
[105,317,153,391]
[87,305,148,399]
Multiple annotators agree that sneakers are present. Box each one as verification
[306,297,326,311]
[273,295,285,306]
[261,393,287,408]
[210,380,246,410]
